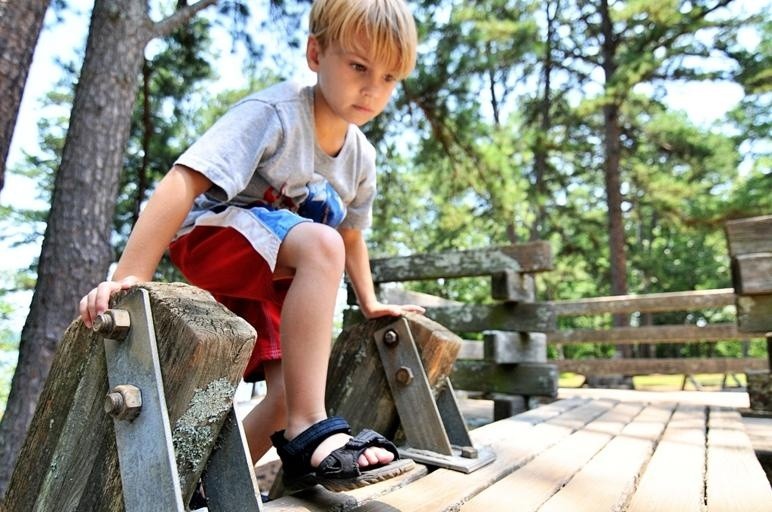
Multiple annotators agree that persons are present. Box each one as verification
[74,0,428,512]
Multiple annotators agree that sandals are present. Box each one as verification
[269,415,417,495]
